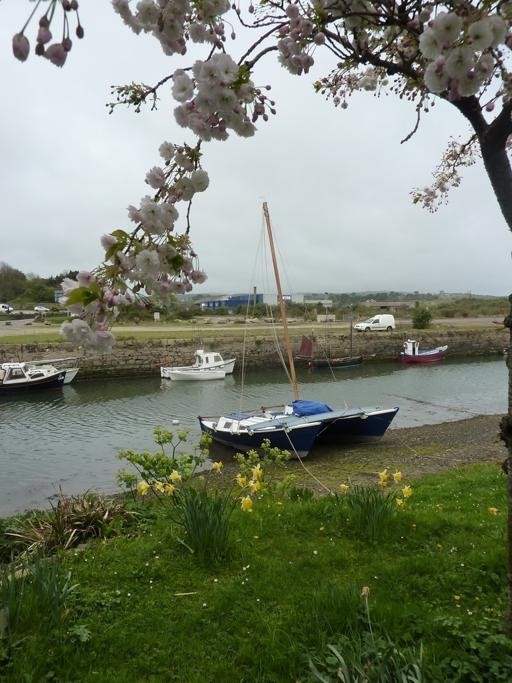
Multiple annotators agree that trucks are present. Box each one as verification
[352,314,396,332]
[0,304,15,314]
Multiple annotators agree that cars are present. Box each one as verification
[34,306,51,314]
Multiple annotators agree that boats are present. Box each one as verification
[160,348,236,377]
[165,368,230,382]
[195,200,398,459]
[0,359,68,397]
[392,338,451,364]
[22,354,87,387]
[296,305,367,370]
